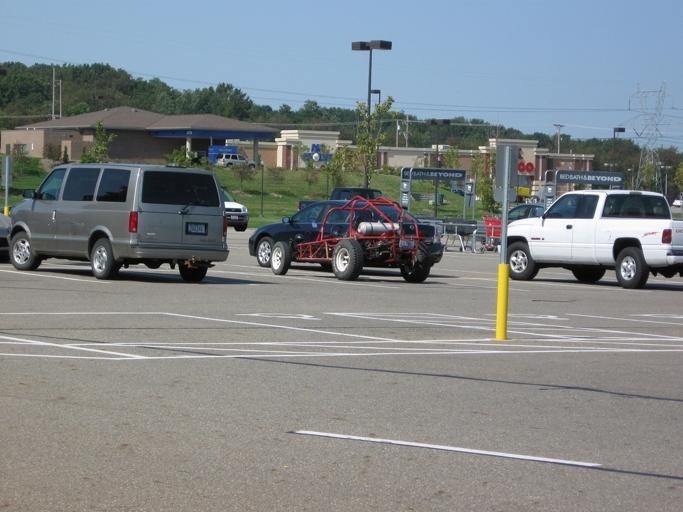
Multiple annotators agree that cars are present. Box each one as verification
[221,188,248,231]
[248,200,445,266]
[0,212,12,264]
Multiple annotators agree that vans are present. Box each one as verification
[5,163,228,283]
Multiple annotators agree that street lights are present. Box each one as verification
[612,127,626,172]
[351,39,392,187]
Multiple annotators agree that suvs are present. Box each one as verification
[216,153,257,170]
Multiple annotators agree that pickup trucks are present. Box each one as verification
[297,187,382,211]
[497,190,682,289]
